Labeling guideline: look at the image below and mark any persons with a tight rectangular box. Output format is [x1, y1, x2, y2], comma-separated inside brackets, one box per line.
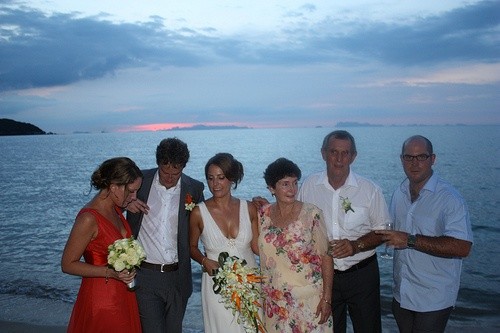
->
[62, 158, 143, 333]
[373, 134, 473, 333]
[188, 153, 264, 333]
[258, 158, 334, 333]
[122, 138, 206, 333]
[253, 130, 391, 333]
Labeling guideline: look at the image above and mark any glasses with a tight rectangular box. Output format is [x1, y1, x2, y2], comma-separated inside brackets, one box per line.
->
[402, 154, 433, 162]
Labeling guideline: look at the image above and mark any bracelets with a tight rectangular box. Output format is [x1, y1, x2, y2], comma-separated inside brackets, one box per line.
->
[324, 299, 332, 305]
[201, 256, 205, 271]
[353, 240, 362, 252]
[105, 269, 109, 282]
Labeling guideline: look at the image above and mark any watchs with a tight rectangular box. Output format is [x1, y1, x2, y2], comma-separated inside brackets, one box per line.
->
[407, 235, 416, 248]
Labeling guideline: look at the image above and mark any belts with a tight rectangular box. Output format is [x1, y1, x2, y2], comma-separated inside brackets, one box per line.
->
[334, 253, 377, 274]
[140, 261, 178, 273]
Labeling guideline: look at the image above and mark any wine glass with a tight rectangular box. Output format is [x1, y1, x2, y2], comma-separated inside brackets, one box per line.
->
[381, 222, 396, 259]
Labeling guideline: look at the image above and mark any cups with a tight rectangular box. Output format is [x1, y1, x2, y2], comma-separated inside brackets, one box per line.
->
[326, 233, 341, 256]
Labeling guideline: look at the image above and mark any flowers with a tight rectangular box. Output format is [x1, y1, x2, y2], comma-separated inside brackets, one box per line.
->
[185, 193, 195, 211]
[339, 196, 355, 214]
[211, 251, 266, 333]
[107, 236, 148, 291]
